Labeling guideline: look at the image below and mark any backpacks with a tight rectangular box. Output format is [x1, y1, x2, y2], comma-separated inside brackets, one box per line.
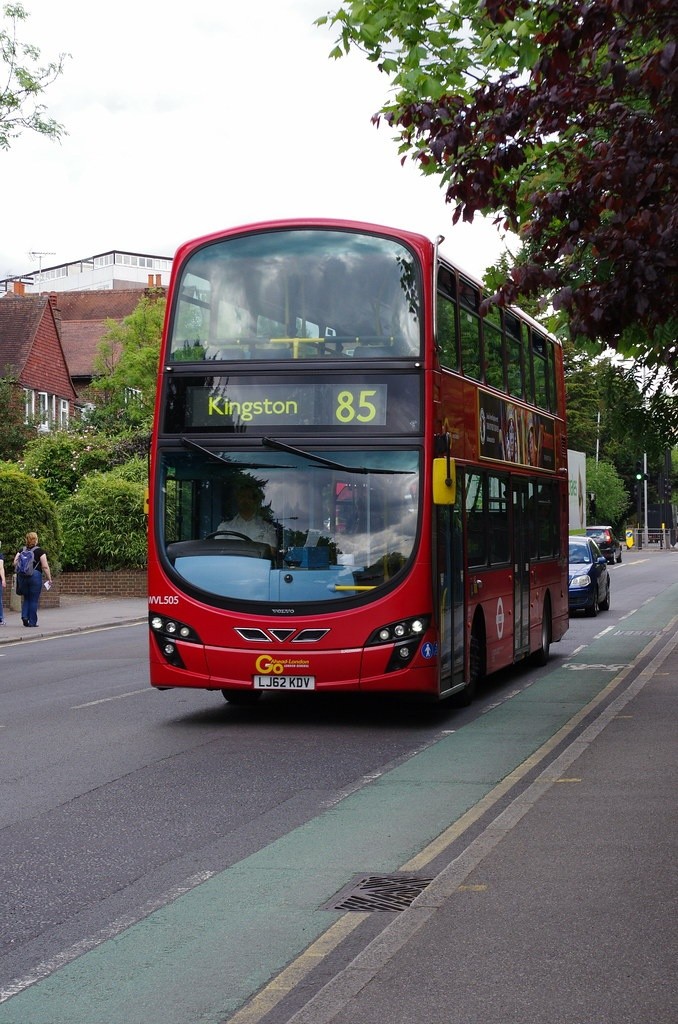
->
[14, 546, 42, 577]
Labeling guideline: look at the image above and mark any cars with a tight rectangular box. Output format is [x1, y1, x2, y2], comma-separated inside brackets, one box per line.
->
[568, 536, 611, 617]
[586, 526, 622, 565]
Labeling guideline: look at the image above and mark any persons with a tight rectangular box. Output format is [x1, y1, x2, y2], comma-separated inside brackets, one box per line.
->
[0, 541, 7, 625]
[528, 423, 536, 466]
[214, 483, 277, 559]
[14, 532, 52, 627]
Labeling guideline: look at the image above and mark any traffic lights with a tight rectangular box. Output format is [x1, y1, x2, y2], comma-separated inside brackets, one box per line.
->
[636, 473, 649, 481]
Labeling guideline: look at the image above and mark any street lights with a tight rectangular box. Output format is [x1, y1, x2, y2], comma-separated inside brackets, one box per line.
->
[590, 409, 613, 526]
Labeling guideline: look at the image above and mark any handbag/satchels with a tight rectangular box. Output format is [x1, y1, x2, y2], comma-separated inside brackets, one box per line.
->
[15, 572, 24, 596]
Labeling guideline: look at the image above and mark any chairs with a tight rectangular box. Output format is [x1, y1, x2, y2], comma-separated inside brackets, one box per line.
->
[214, 332, 409, 357]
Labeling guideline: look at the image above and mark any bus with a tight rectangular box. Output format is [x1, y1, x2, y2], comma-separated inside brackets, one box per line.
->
[147, 218, 568, 728]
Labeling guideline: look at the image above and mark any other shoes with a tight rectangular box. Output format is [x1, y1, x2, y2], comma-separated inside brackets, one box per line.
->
[23, 618, 28, 626]
[0, 622, 6, 627]
[30, 623, 39, 627]
